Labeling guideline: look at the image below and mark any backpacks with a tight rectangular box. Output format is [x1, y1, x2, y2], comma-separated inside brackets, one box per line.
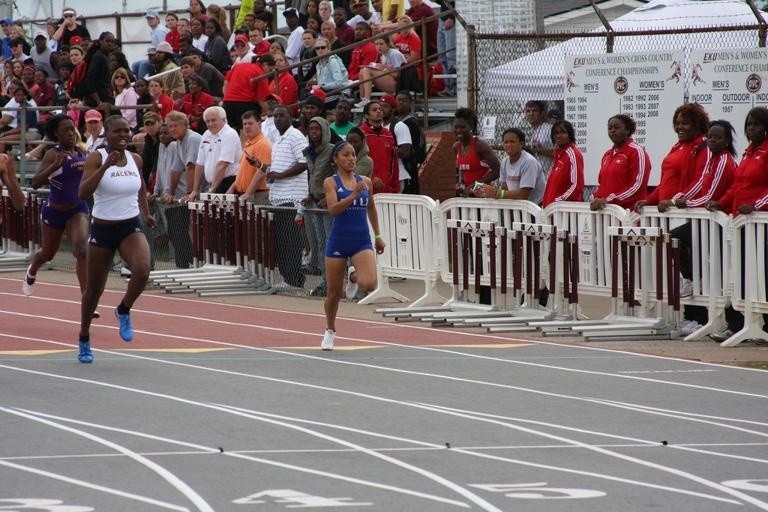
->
[403, 113, 427, 163]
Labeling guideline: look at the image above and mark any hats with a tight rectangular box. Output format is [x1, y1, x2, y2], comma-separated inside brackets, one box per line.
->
[63, 8, 76, 16]
[145, 41, 174, 60]
[303, 87, 327, 104]
[235, 35, 249, 46]
[143, 109, 161, 126]
[264, 93, 283, 108]
[234, 25, 249, 33]
[282, 6, 300, 21]
[84, 109, 103, 123]
[32, 30, 47, 41]
[144, 9, 159, 19]
[379, 94, 399, 110]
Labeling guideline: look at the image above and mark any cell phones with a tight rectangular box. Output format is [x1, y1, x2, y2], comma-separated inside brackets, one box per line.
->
[247, 157, 256, 164]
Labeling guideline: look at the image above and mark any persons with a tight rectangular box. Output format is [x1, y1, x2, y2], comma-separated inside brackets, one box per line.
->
[78, 115, 158, 361]
[21, 115, 102, 318]
[450, 99, 767, 346]
[316, 141, 387, 349]
[1, 0, 455, 296]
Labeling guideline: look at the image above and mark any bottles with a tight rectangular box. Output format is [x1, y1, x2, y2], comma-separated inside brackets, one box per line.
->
[294, 202, 305, 224]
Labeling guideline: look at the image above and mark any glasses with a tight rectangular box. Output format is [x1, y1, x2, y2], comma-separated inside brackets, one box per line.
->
[65, 14, 73, 18]
[114, 75, 125, 79]
[315, 45, 328, 50]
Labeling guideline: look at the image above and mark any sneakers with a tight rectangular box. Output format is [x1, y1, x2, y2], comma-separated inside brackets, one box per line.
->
[679, 278, 701, 299]
[345, 265, 360, 300]
[22, 264, 37, 295]
[271, 280, 304, 292]
[672, 318, 745, 348]
[320, 327, 336, 351]
[299, 261, 321, 277]
[77, 335, 94, 364]
[114, 304, 134, 342]
[119, 266, 132, 276]
[354, 96, 371, 111]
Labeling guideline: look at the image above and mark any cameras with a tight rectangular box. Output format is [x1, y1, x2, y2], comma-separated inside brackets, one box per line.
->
[473, 183, 483, 192]
